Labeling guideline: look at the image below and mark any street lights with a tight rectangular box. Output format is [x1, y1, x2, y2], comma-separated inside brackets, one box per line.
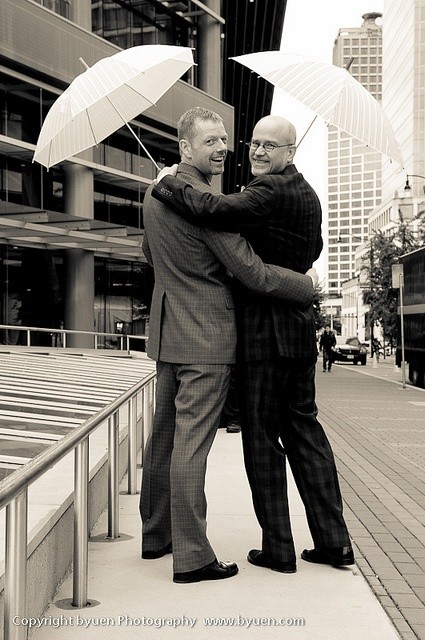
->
[337, 232, 374, 359]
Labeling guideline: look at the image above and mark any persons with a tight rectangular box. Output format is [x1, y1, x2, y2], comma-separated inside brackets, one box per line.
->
[139, 107, 324, 583]
[150, 114, 355, 574]
[319, 320, 337, 373]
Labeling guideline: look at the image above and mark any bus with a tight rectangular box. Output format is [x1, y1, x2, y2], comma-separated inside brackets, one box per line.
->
[393, 246, 424, 384]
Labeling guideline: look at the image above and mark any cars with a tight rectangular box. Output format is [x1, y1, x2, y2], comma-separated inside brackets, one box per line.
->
[332, 334, 368, 366]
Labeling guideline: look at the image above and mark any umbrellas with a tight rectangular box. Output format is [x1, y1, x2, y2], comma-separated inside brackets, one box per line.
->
[228, 45, 412, 172]
[29, 43, 198, 174]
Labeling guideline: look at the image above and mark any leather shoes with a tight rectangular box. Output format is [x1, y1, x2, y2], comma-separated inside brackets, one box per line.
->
[227, 422, 240, 432]
[247, 549, 296, 573]
[173, 560, 239, 583]
[301, 545, 354, 565]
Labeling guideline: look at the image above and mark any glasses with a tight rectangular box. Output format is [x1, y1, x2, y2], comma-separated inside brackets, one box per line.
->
[243, 142, 294, 151]
